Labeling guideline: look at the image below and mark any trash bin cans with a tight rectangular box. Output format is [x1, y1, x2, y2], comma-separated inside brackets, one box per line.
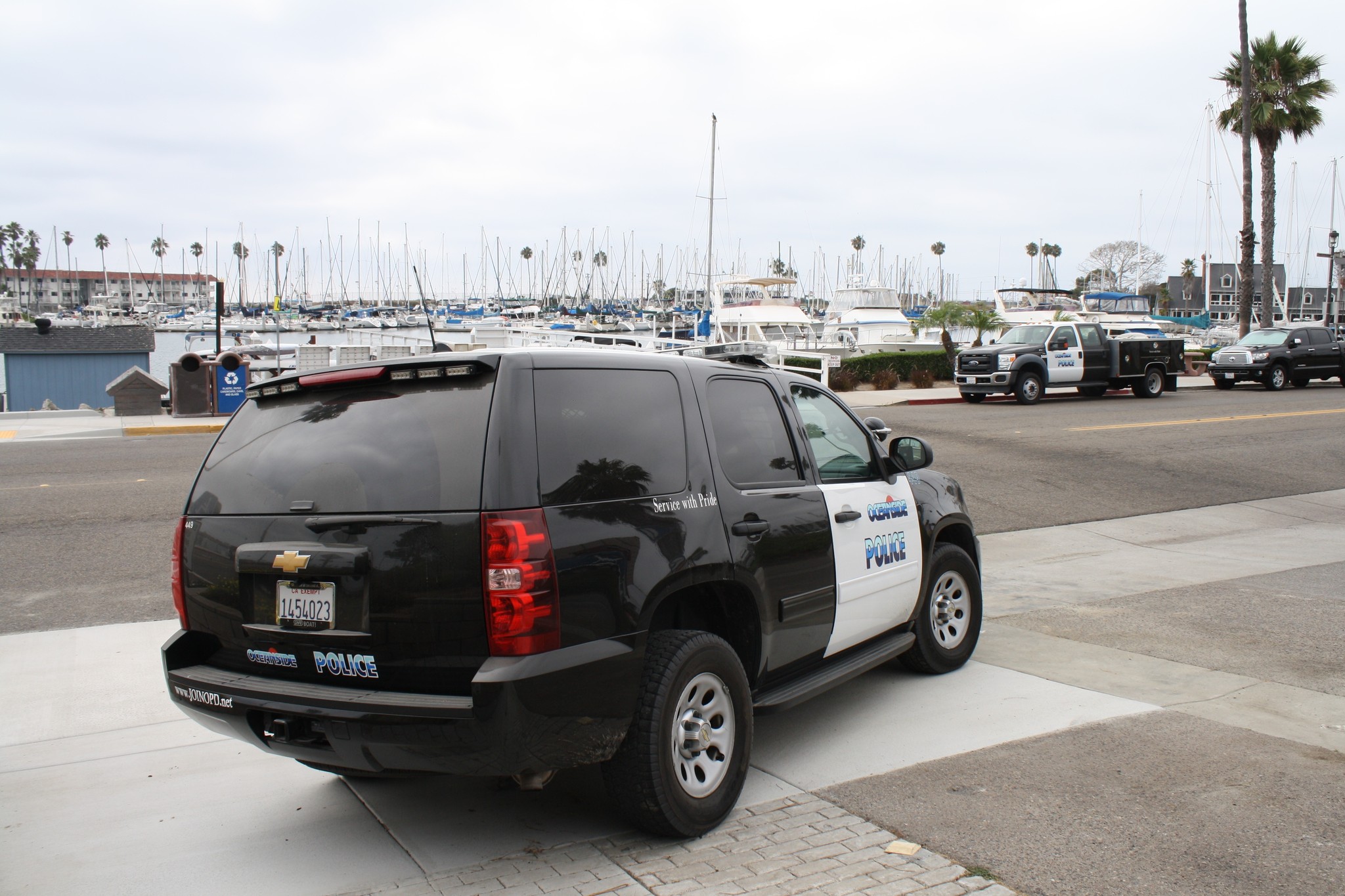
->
[210, 350, 251, 417]
[168, 352, 213, 418]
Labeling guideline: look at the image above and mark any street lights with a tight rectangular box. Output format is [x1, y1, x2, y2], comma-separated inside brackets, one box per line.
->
[1325, 230, 1340, 327]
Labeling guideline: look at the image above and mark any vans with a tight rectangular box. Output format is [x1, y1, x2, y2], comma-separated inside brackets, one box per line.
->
[160, 266, 983, 839]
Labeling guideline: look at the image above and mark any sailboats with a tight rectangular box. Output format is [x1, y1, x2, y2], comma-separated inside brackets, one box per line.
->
[0, 113, 957, 364]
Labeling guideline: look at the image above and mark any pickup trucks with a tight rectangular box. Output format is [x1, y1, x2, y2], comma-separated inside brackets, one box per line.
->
[1208, 327, 1345, 391]
[954, 321, 1189, 406]
